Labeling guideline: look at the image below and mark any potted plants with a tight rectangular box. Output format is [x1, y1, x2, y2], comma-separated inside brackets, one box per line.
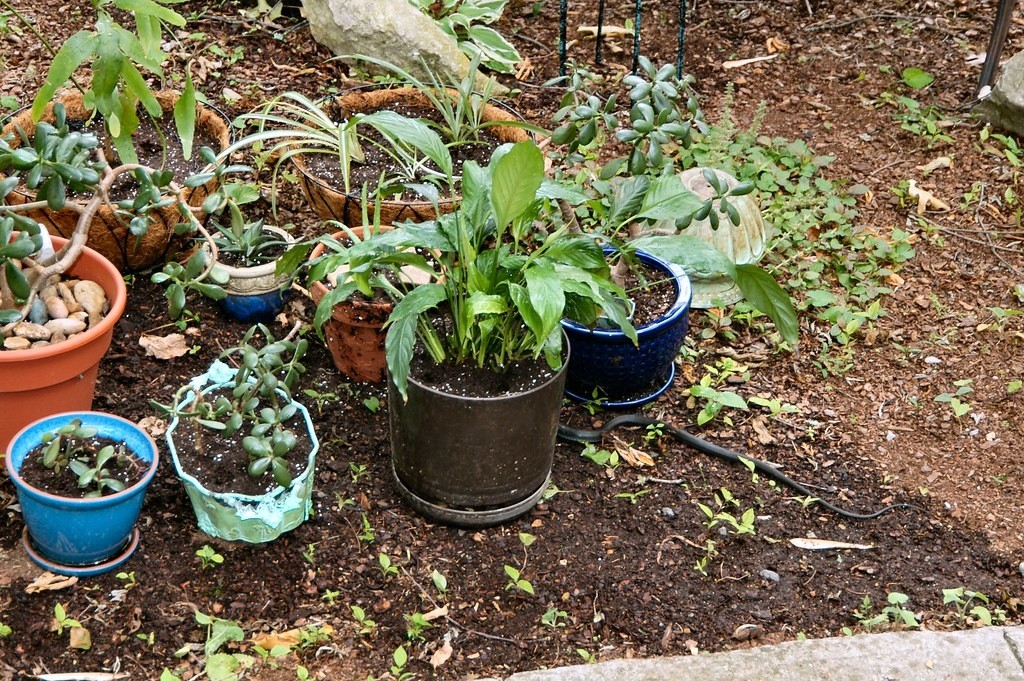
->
[192, 218, 306, 320]
[6, 410, 160, 577]
[197, 47, 552, 233]
[308, 138, 651, 528]
[306, 170, 448, 383]
[548, 52, 758, 414]
[148, 320, 321, 544]
[0, 1, 258, 339]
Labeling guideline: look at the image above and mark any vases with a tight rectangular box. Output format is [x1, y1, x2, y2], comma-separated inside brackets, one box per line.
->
[0, 229, 127, 463]
